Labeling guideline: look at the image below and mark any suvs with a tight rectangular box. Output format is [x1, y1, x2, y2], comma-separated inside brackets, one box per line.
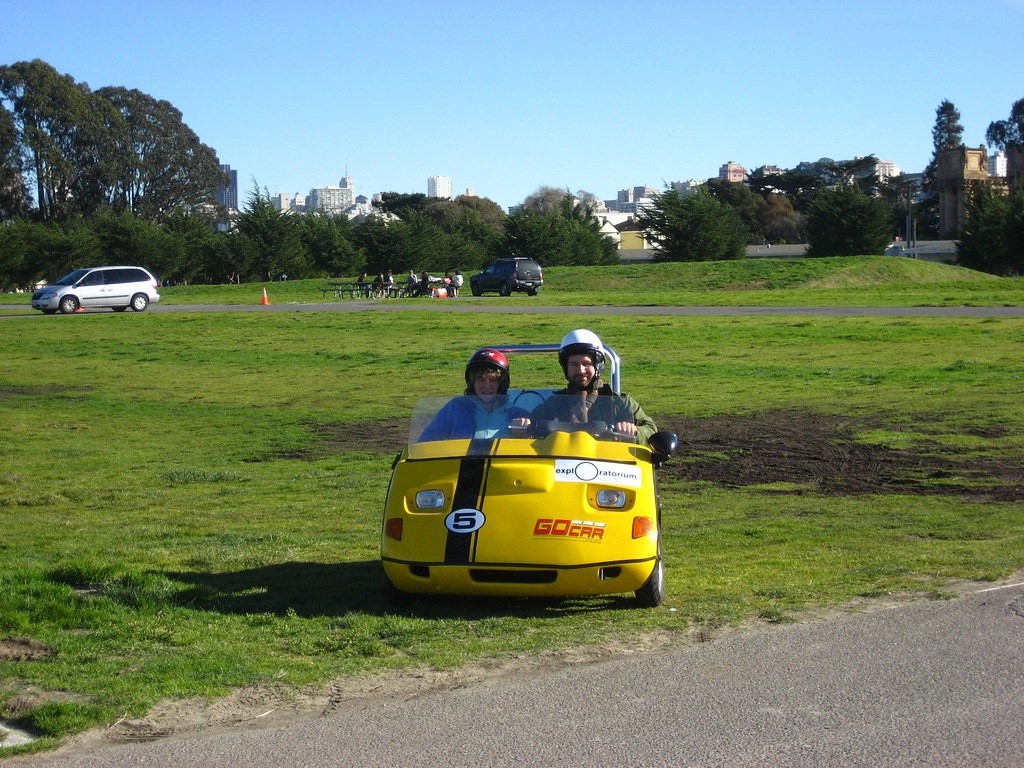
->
[469, 257, 544, 297]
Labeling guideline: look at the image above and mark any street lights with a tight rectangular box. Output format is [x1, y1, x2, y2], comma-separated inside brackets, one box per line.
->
[260, 287, 270, 306]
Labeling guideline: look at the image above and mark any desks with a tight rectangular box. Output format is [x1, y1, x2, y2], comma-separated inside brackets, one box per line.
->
[332, 282, 370, 299]
[397, 281, 442, 298]
[355, 282, 392, 298]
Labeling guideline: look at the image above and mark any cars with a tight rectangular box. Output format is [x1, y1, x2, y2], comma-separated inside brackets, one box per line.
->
[32, 265, 161, 314]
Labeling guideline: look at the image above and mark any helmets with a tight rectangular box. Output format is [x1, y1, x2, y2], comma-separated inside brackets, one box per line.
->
[466, 347, 509, 371]
[559, 329, 605, 363]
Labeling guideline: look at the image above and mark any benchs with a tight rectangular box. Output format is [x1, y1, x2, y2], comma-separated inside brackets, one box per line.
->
[322, 280, 458, 299]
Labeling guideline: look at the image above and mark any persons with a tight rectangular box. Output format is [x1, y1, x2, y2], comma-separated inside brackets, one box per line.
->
[417, 348, 531, 442]
[511, 329, 657, 446]
[358, 269, 463, 299]
